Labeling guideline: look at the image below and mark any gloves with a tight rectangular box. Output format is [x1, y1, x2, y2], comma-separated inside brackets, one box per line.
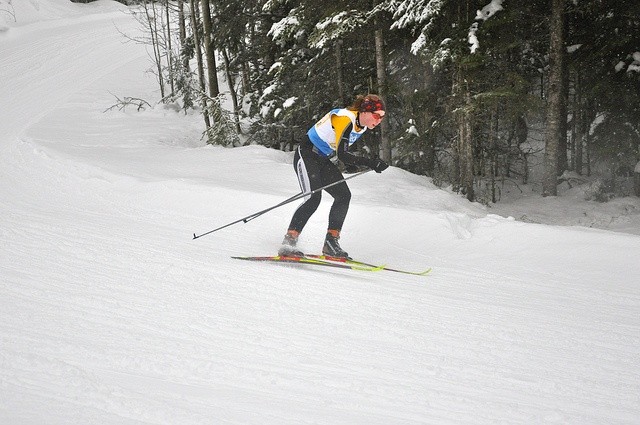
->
[370, 158, 389, 173]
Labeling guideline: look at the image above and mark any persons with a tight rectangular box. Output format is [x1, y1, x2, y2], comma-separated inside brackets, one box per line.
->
[279, 93, 390, 259]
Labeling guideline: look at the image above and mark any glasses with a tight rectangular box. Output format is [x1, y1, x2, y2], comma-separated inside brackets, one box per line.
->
[371, 110, 385, 119]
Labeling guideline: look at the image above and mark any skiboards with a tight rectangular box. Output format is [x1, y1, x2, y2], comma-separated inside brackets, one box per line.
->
[231, 251, 431, 276]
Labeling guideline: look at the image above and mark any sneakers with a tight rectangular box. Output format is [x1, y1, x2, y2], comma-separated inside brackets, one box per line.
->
[278, 234, 304, 257]
[322, 232, 348, 257]
